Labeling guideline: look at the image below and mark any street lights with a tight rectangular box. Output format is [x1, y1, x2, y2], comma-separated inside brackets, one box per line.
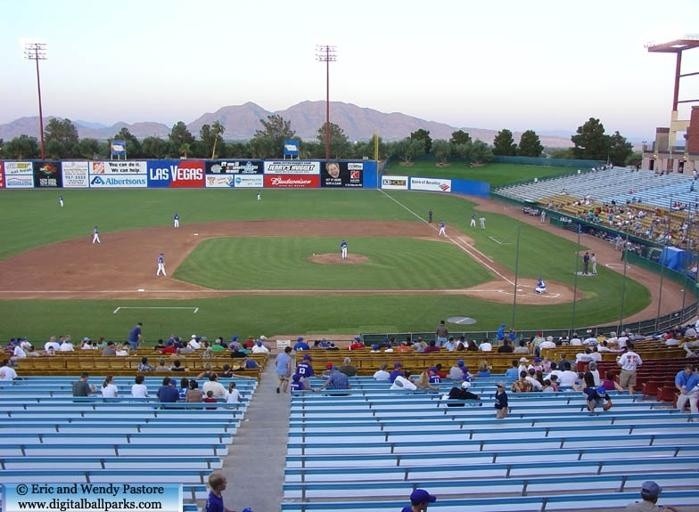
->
[607, 144, 614, 163]
[23, 40, 48, 159]
[315, 44, 338, 158]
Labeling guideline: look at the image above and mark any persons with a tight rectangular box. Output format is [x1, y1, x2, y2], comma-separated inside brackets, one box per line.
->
[58, 195, 65, 207]
[469, 214, 477, 227]
[92, 225, 102, 245]
[437, 220, 447, 238]
[172, 212, 181, 229]
[325, 162, 340, 179]
[521, 167, 699, 276]
[400, 488, 437, 511]
[156, 252, 168, 277]
[340, 239, 349, 260]
[535, 277, 546, 294]
[478, 216, 487, 231]
[626, 480, 676, 511]
[203, 473, 240, 511]
[255, 190, 262, 201]
[427, 207, 434, 224]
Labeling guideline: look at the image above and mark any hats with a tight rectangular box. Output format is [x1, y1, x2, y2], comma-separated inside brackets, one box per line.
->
[461, 381, 471, 389]
[293, 374, 301, 379]
[326, 362, 333, 369]
[640, 480, 662, 496]
[304, 354, 312, 361]
[596, 387, 606, 398]
[458, 360, 464, 367]
[409, 489, 437, 502]
[394, 362, 403, 369]
[520, 357, 529, 363]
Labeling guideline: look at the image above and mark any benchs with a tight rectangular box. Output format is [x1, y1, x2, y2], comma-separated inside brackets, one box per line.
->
[490, 164, 699, 258]
[1, 332, 699, 511]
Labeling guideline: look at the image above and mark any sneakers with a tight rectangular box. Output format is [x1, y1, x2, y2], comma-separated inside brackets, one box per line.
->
[277, 387, 280, 394]
